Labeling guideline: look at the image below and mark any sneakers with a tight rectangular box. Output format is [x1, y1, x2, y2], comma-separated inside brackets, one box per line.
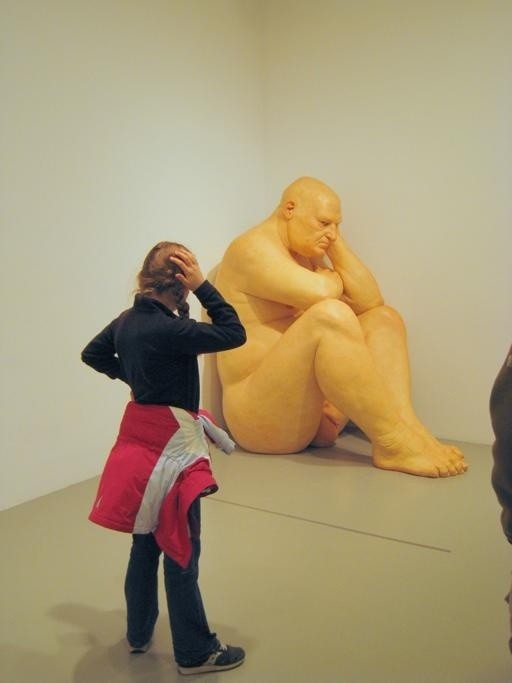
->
[127, 636, 154, 653]
[178, 644, 245, 675]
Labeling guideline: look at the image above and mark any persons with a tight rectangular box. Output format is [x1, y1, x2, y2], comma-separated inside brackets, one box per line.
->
[216, 176, 468, 477]
[489, 347, 512, 655]
[79, 241, 246, 677]
